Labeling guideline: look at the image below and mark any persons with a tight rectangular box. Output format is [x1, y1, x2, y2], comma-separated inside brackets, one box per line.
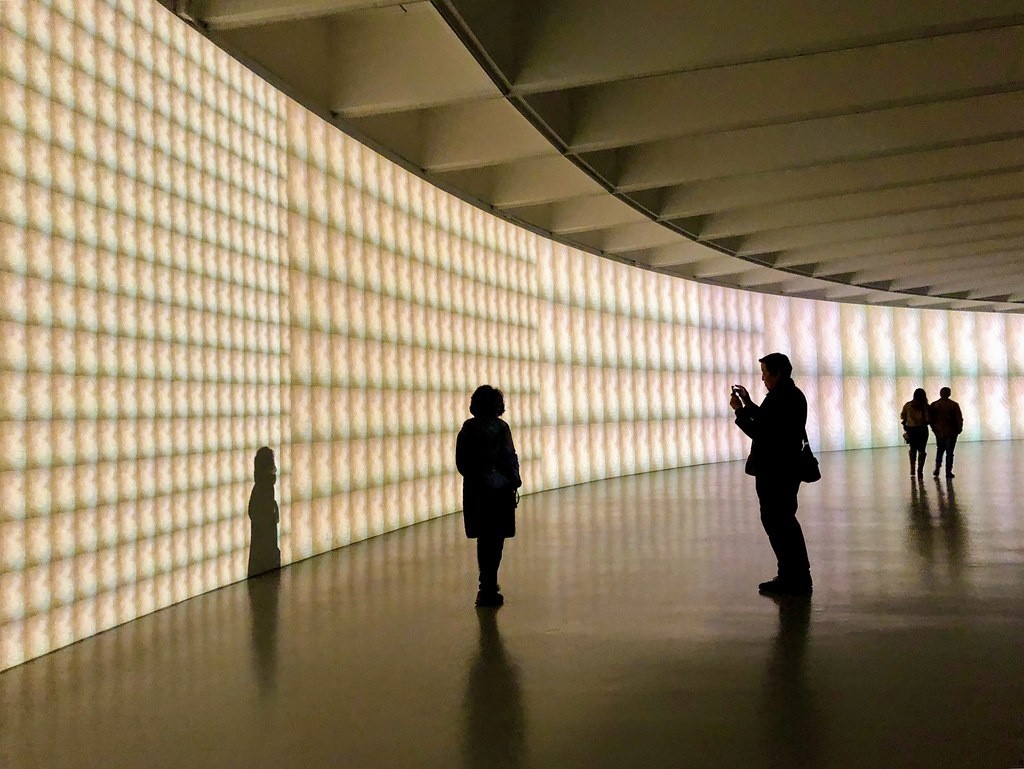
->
[928, 386, 964, 479]
[456, 384, 523, 611]
[900, 388, 930, 478]
[730, 352, 813, 596]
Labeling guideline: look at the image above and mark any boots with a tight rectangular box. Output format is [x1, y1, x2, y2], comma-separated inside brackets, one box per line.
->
[909, 450, 916, 475]
[918, 453, 926, 478]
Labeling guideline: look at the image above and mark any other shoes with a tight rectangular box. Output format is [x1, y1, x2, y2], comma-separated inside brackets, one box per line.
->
[934, 470, 939, 476]
[759, 576, 812, 594]
[946, 473, 955, 478]
[474, 593, 503, 606]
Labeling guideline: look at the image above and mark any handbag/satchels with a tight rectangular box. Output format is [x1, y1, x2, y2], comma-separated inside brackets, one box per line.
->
[802, 444, 821, 482]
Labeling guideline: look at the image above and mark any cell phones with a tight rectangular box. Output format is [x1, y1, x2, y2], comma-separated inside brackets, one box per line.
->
[731, 386, 736, 395]
